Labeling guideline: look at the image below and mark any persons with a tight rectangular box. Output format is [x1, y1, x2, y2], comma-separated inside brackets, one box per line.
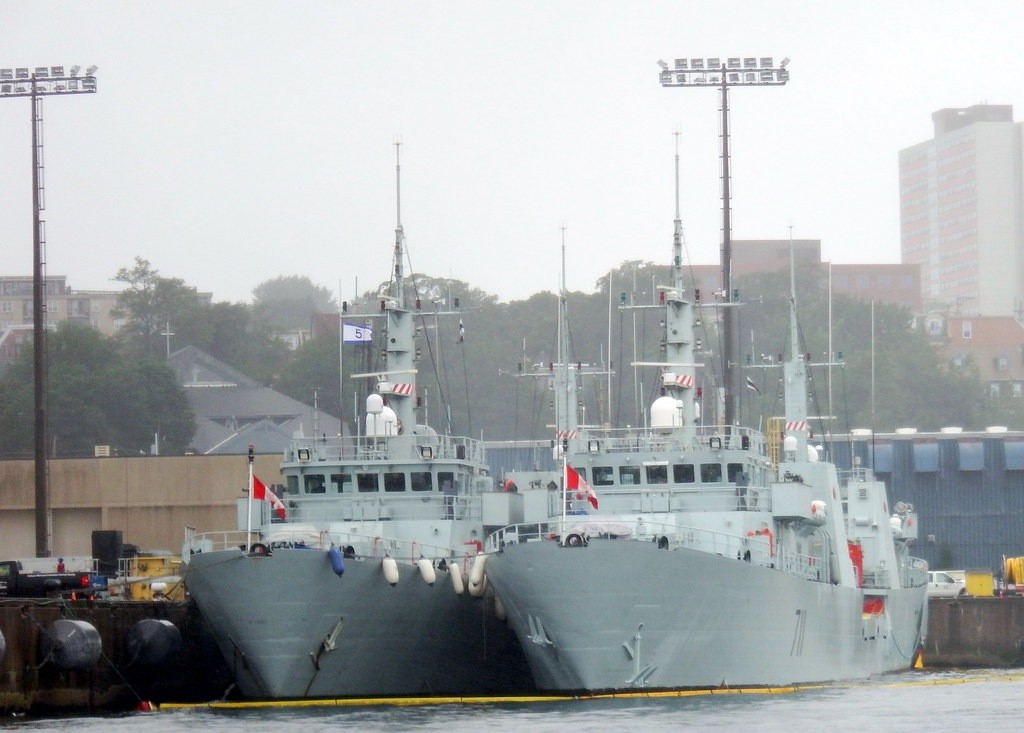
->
[496, 476, 514, 492]
[464, 530, 482, 554]
[57, 557, 65, 575]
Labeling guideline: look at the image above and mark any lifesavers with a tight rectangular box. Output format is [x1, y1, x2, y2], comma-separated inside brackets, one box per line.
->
[566, 534, 582, 546]
[745, 550, 751, 561]
[658, 536, 669, 550]
[344, 546, 355, 558]
[249, 543, 268, 557]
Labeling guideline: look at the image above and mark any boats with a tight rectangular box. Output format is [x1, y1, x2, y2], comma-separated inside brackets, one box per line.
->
[179, 127, 532, 710]
[481, 125, 931, 704]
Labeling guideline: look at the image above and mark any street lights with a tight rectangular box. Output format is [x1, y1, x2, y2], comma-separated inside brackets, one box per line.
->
[658, 56, 793, 441]
[0, 61, 98, 562]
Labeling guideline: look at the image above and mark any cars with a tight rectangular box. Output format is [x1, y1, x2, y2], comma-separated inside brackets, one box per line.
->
[946, 568, 1024, 597]
[927, 570, 970, 597]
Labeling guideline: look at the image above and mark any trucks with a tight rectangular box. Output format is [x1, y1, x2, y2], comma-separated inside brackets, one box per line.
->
[0, 560, 98, 604]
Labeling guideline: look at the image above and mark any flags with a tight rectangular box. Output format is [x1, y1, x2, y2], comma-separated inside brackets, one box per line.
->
[343, 321, 374, 345]
[253, 476, 286, 521]
[567, 466, 599, 510]
